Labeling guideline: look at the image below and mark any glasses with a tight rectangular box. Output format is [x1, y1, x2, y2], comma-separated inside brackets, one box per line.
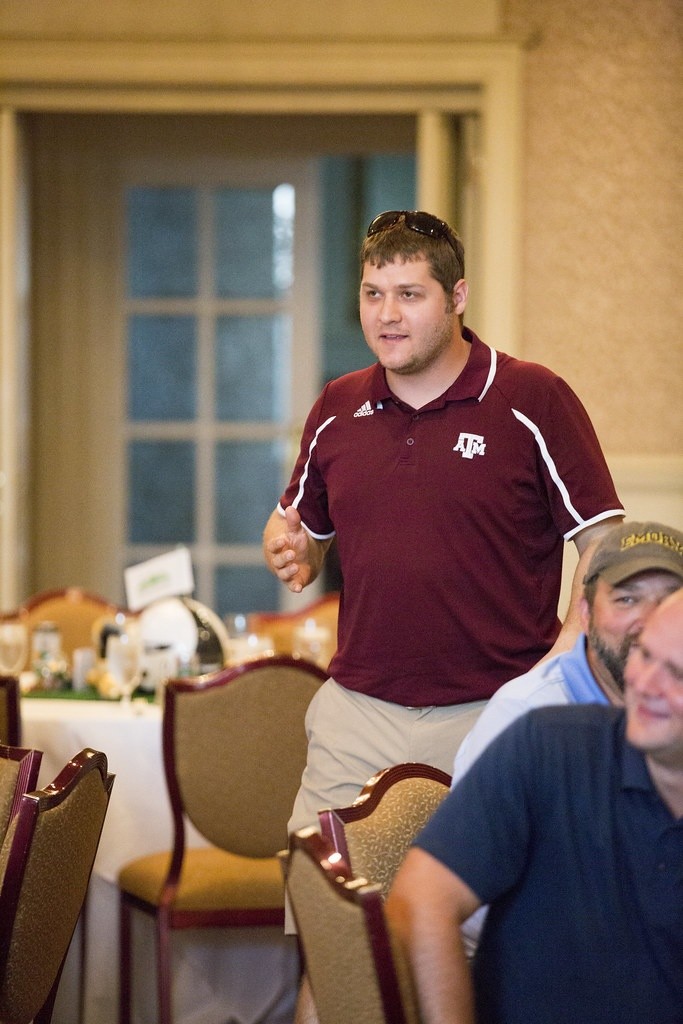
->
[366, 209, 460, 265]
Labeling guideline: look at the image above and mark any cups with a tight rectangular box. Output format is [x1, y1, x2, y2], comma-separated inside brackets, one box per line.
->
[0, 624, 29, 677]
[72, 649, 95, 692]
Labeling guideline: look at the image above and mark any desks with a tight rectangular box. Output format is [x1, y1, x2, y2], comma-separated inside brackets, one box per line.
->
[14, 696, 299, 1024]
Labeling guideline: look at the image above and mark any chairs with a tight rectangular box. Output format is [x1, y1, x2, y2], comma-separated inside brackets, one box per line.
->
[0, 588, 452, 1024]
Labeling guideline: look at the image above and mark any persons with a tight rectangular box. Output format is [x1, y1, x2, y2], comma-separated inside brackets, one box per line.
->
[260, 210, 629, 1024]
[447, 522, 683, 958]
[384, 587, 683, 1024]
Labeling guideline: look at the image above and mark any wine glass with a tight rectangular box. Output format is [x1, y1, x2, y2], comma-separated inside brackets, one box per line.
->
[105, 636, 143, 709]
[31, 623, 63, 689]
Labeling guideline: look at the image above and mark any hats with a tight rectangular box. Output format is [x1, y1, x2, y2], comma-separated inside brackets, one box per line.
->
[582, 521, 683, 588]
